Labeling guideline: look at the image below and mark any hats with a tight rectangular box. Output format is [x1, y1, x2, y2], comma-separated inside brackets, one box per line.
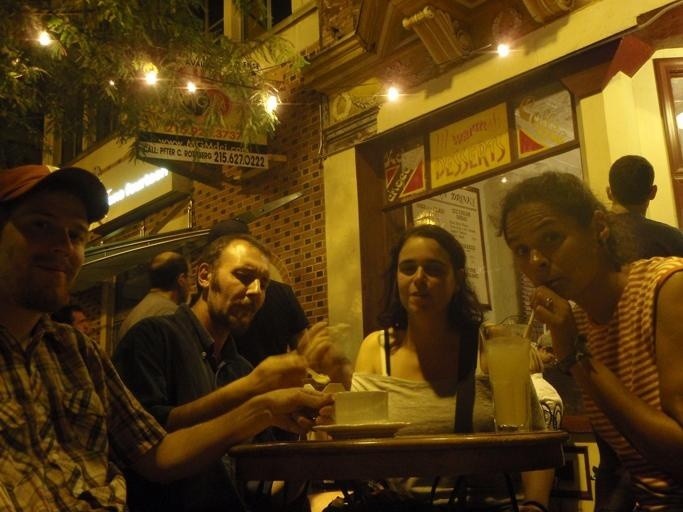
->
[0, 164, 108, 223]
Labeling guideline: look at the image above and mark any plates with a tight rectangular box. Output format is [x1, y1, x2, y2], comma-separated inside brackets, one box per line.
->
[312, 423, 411, 440]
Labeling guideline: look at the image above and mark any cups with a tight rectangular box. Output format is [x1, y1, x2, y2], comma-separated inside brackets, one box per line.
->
[480, 325, 535, 433]
[333, 391, 388, 424]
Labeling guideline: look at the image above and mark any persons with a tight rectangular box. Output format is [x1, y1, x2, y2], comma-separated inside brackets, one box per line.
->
[605, 156, 682, 272]
[349, 226, 556, 512]
[495, 171, 682, 512]
[50, 220, 357, 512]
[2, 165, 336, 512]
